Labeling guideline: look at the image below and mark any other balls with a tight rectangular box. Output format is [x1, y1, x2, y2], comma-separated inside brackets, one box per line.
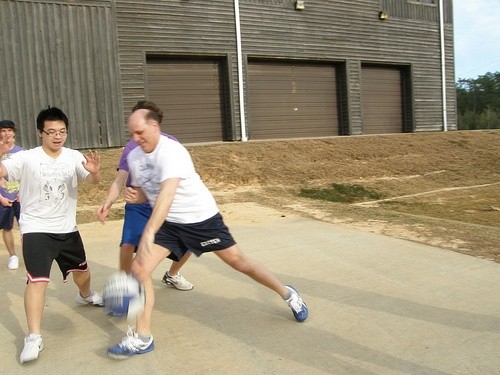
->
[101, 270, 148, 322]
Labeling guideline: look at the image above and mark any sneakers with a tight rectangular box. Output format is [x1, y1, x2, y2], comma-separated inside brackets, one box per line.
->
[162, 271, 194, 290]
[76, 291, 104, 307]
[284, 285, 309, 322]
[20, 334, 44, 363]
[107, 324, 155, 359]
[8, 256, 19, 270]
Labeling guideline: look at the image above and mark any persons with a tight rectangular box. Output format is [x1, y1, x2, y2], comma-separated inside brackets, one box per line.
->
[0, 107, 101, 366]
[97, 101, 308, 361]
[0, 120, 26, 270]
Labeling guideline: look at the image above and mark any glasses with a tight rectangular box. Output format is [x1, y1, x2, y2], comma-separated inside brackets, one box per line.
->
[42, 129, 67, 138]
